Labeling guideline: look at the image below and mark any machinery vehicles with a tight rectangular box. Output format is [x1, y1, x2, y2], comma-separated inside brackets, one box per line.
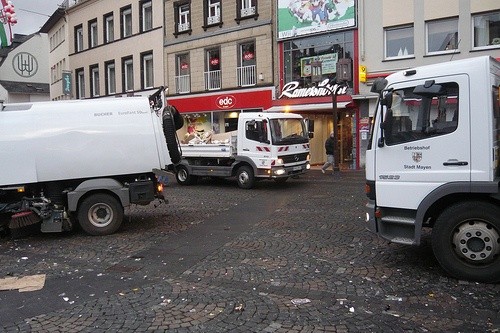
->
[0, 86, 184, 236]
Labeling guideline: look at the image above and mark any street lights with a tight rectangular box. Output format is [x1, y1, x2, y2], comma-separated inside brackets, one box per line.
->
[310, 58, 351, 179]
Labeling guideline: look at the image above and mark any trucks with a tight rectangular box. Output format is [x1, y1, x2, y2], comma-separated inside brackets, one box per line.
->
[363, 55, 500, 283]
[177, 112, 314, 190]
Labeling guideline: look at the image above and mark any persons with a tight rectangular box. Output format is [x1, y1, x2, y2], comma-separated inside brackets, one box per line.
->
[321, 131, 336, 175]
